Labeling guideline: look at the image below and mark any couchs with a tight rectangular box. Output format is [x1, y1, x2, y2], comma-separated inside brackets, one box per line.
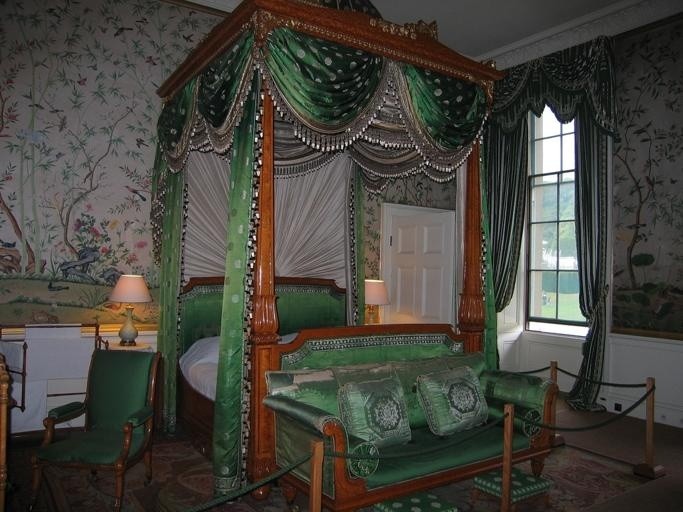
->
[262, 321, 559, 512]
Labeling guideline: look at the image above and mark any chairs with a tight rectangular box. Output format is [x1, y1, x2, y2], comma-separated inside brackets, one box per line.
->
[24, 347, 162, 511]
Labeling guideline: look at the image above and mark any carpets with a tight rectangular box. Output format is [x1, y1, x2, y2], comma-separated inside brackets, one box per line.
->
[43, 439, 648, 512]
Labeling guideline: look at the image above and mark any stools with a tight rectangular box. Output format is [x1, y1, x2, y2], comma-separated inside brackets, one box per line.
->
[370, 466, 553, 512]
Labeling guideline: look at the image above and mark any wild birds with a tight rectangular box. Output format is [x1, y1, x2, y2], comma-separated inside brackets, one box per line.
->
[0, 239, 18, 248]
[135, 137, 151, 149]
[145, 55, 158, 67]
[624, 223, 647, 231]
[113, 18, 149, 39]
[183, 34, 195, 44]
[123, 184, 147, 203]
[632, 177, 658, 201]
[48, 283, 69, 291]
[20, 89, 66, 114]
[663, 283, 683, 305]
[77, 73, 88, 86]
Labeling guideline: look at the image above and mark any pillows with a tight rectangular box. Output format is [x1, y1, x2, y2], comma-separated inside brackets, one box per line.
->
[265, 351, 488, 449]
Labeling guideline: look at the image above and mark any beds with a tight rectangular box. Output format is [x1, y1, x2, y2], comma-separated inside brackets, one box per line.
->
[176, 275, 464, 500]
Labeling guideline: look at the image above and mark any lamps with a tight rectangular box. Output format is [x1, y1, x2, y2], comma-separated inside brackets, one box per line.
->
[109, 273, 153, 346]
[364, 279, 390, 324]
